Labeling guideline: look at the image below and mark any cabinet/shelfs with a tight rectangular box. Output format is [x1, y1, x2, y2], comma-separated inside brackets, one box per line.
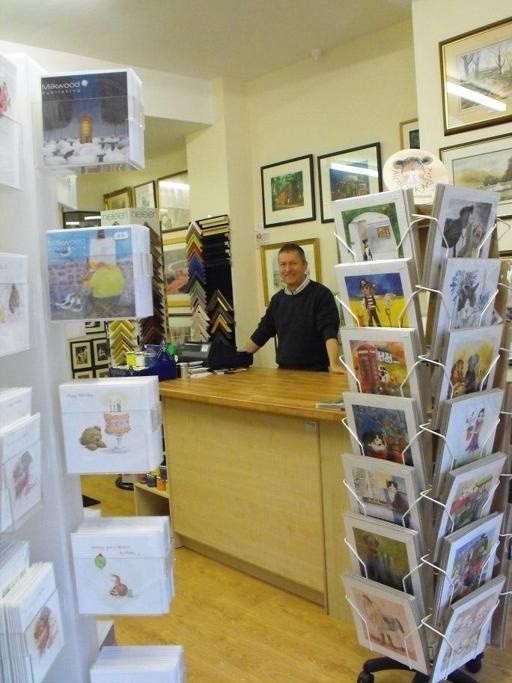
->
[130, 401, 185, 550]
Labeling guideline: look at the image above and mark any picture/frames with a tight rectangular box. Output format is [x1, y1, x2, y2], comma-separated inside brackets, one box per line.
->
[438, 132, 511, 221]
[438, 15, 512, 137]
[168, 312, 194, 345]
[162, 237, 191, 306]
[399, 117, 418, 151]
[259, 152, 315, 228]
[70, 320, 111, 381]
[133, 181, 156, 209]
[156, 169, 195, 234]
[259, 238, 322, 307]
[316, 141, 383, 224]
[102, 185, 132, 210]
[496, 250, 512, 368]
